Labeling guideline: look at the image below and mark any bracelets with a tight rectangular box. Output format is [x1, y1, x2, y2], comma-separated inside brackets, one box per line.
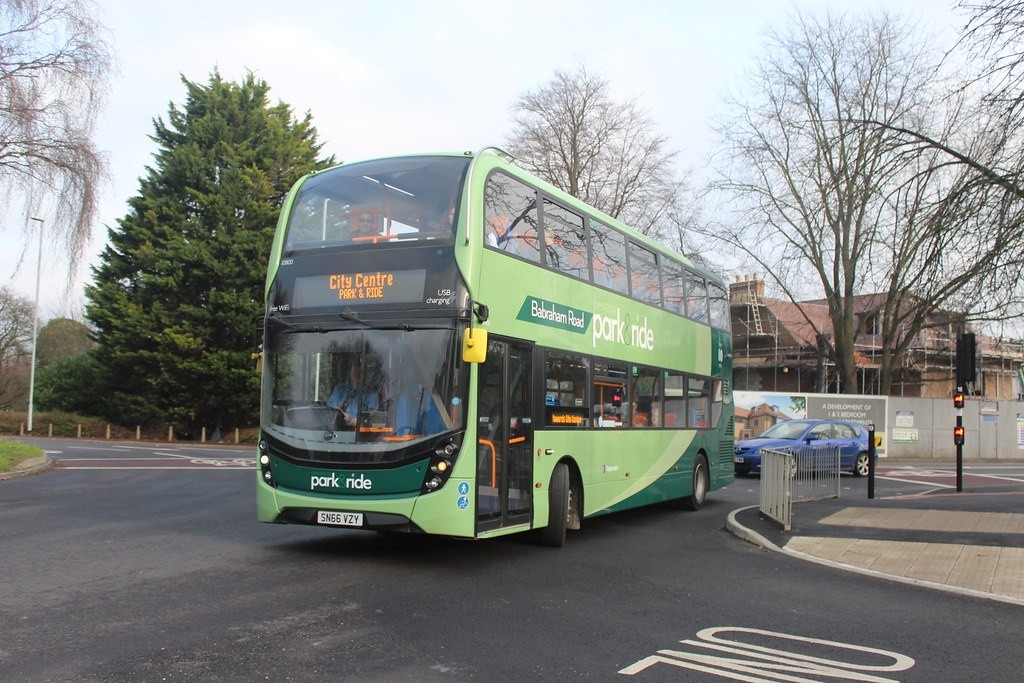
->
[346, 414, 353, 425]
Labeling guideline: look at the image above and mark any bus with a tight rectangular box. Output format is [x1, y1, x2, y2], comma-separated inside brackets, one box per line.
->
[251, 146, 734, 547]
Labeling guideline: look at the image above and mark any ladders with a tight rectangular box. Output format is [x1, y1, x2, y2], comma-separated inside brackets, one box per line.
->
[769, 314, 786, 362]
[750, 291, 762, 333]
[966, 382, 975, 396]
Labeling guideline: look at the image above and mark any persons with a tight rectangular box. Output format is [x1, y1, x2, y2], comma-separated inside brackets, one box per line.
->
[348, 212, 387, 243]
[328, 360, 380, 432]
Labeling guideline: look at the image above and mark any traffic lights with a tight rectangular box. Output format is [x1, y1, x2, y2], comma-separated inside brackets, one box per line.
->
[954, 427, 963, 446]
[954, 394, 964, 408]
[612, 390, 621, 407]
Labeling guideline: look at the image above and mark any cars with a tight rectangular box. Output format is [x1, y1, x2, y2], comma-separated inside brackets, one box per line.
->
[734, 419, 878, 479]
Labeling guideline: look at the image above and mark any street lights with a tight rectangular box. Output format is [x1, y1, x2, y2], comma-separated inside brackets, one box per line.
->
[25, 217, 44, 433]
